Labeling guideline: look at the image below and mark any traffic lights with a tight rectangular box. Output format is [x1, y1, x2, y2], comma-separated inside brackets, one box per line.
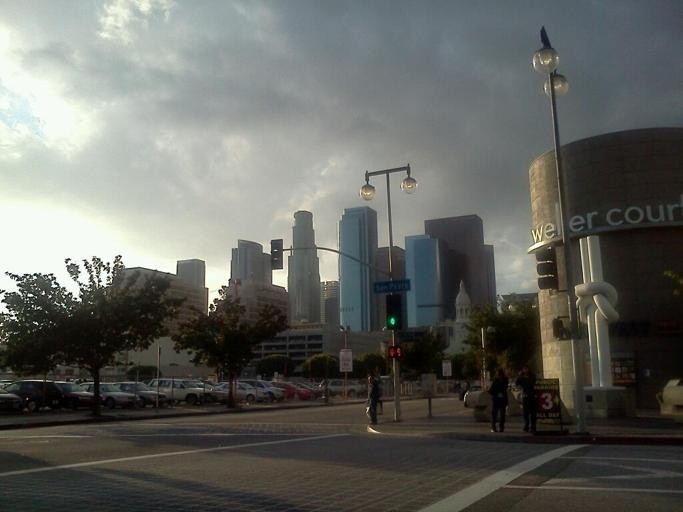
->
[557, 327, 571, 340]
[385, 293, 402, 331]
[388, 345, 403, 359]
[270, 237, 284, 271]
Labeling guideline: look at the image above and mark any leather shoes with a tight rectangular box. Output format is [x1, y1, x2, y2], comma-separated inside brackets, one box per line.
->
[498, 423, 503, 432]
[490, 424, 496, 432]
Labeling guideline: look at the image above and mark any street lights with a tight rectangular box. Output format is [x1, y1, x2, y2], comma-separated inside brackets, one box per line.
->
[531, 24, 590, 443]
[339, 324, 350, 399]
[358, 162, 418, 424]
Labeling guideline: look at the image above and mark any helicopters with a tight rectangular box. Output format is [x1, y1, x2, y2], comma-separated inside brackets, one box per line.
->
[534, 244, 560, 292]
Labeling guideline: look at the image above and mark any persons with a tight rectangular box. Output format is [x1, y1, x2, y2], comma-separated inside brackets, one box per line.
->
[515, 363, 537, 432]
[486, 367, 509, 432]
[463, 378, 469, 392]
[368, 375, 381, 425]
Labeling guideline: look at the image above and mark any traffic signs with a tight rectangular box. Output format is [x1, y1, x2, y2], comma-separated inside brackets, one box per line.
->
[373, 279, 411, 293]
